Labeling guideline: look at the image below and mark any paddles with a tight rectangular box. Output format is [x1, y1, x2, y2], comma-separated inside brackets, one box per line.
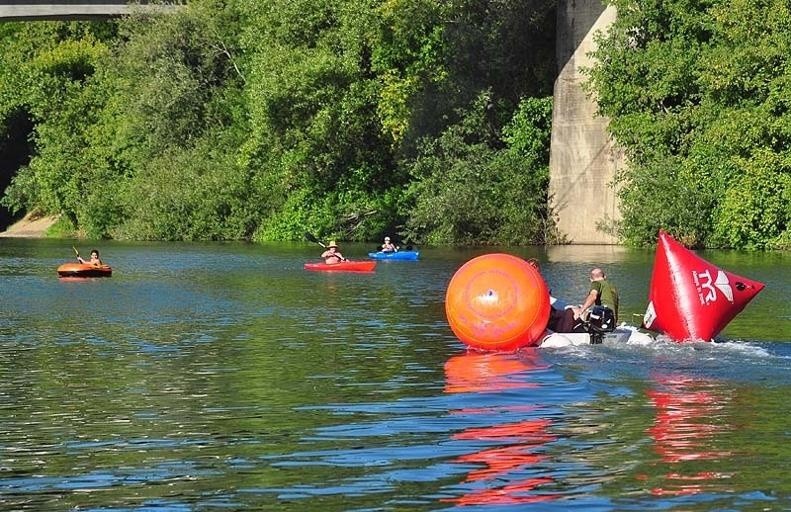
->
[317, 242, 350, 262]
[73, 246, 82, 264]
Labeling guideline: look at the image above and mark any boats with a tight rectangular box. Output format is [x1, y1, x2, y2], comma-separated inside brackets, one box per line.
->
[304, 260, 377, 272]
[58, 260, 113, 279]
[534, 294, 657, 351]
[370, 249, 420, 262]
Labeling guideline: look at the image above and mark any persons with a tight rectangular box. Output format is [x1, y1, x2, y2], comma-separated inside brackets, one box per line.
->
[559, 267, 620, 333]
[382, 236, 398, 252]
[78, 250, 102, 266]
[321, 241, 349, 264]
[529, 256, 566, 333]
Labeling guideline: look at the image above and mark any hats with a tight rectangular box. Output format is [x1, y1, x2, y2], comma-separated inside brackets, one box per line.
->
[326, 241, 338, 248]
[384, 236, 390, 241]
